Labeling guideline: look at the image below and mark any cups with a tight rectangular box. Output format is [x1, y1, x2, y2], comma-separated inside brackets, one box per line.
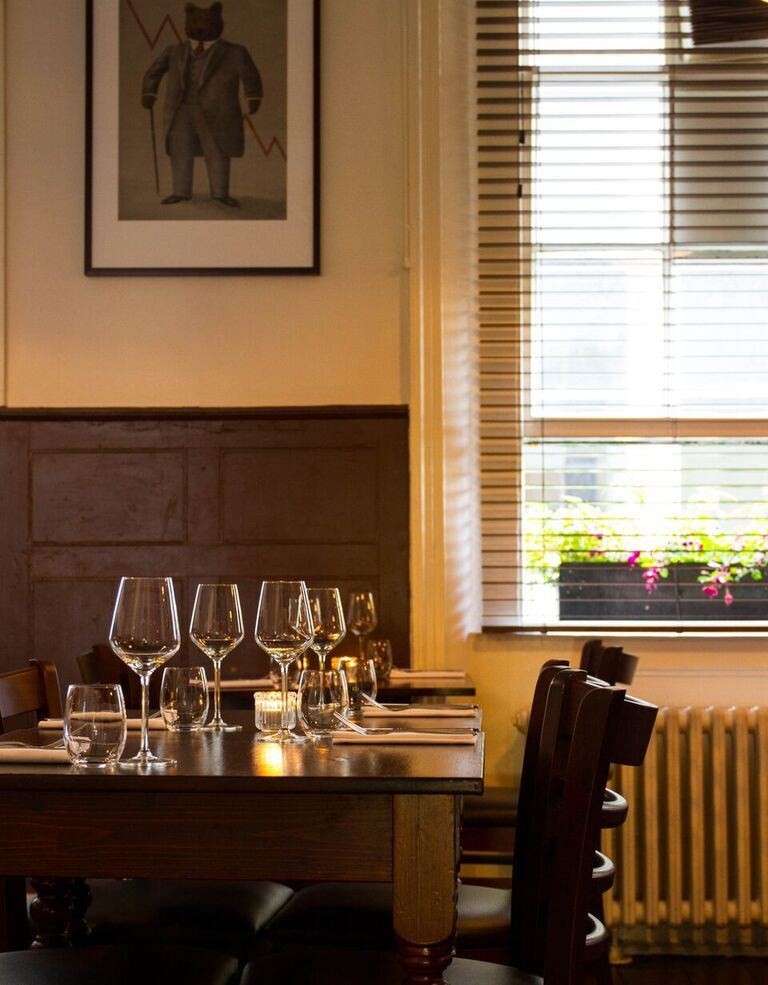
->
[367, 638, 393, 678]
[253, 691, 298, 735]
[63, 684, 127, 768]
[159, 666, 210, 733]
[337, 658, 378, 706]
[296, 668, 349, 735]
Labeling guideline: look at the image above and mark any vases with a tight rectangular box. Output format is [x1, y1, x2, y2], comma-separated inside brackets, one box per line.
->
[559, 561, 768, 620]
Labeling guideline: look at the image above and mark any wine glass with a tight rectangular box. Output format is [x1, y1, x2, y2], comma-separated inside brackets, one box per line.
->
[347, 592, 377, 661]
[254, 581, 315, 744]
[188, 583, 245, 732]
[296, 588, 347, 712]
[108, 575, 181, 769]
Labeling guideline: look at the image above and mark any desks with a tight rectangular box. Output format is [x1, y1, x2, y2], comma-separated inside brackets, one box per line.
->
[181, 670, 476, 705]
[0, 704, 485, 985]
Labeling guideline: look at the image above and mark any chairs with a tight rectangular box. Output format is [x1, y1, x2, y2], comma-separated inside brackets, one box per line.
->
[0, 642, 658, 985]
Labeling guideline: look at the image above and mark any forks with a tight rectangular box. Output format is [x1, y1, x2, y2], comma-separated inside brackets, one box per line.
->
[0, 723, 83, 749]
[332, 693, 473, 736]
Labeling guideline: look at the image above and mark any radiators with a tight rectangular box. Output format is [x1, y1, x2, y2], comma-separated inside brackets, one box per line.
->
[516, 703, 768, 964]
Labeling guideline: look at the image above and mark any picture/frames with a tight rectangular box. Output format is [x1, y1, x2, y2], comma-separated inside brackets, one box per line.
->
[83, 0, 321, 278]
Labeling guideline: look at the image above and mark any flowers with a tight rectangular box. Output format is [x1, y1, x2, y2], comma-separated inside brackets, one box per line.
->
[528, 490, 768, 606]
